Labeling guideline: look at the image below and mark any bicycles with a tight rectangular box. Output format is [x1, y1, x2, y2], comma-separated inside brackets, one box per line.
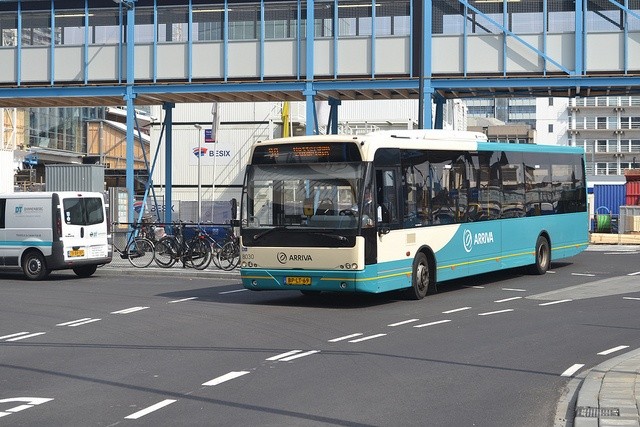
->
[140, 217, 241, 272]
[97, 220, 156, 268]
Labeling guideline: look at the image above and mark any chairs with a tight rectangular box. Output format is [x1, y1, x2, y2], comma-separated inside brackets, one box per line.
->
[406, 183, 563, 225]
[315, 198, 334, 214]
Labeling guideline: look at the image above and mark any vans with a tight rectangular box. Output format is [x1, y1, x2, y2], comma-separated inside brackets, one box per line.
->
[1, 190, 113, 281]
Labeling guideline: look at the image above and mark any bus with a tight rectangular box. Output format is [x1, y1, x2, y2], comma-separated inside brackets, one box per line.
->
[238, 128, 591, 300]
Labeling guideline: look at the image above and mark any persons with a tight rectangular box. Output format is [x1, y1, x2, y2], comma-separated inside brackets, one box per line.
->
[352, 188, 383, 222]
